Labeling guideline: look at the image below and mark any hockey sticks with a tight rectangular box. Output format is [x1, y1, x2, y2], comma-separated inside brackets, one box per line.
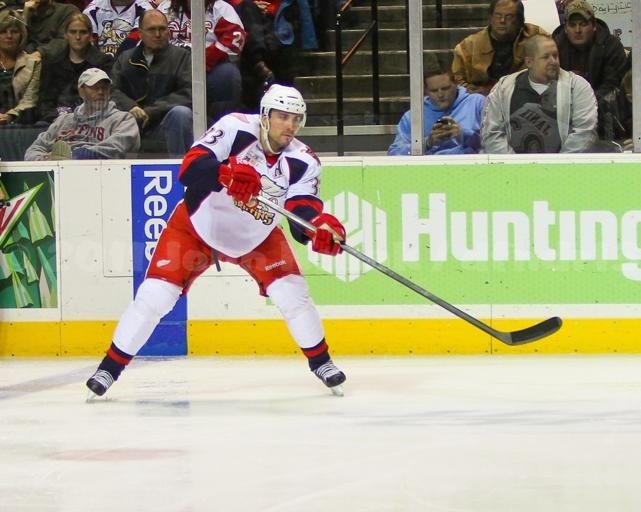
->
[258, 197, 562, 345]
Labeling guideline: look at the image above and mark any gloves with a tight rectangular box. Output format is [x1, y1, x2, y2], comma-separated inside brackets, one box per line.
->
[305, 213, 345, 256]
[218, 156, 261, 208]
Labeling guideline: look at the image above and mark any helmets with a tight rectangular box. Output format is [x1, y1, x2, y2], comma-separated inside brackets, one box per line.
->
[260, 84, 307, 132]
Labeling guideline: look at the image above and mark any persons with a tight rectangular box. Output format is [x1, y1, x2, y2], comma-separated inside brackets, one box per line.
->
[0, 0, 348, 159]
[80, 84, 346, 357]
[385, 0, 633, 153]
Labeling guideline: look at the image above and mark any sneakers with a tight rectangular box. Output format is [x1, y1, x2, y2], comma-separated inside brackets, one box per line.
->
[313, 359, 346, 387]
[87, 369, 114, 395]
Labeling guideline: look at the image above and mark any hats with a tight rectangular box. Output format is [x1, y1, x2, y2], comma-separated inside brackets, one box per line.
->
[77, 68, 112, 88]
[564, 0, 594, 21]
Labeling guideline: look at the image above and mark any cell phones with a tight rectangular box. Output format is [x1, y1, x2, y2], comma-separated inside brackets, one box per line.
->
[437, 119, 449, 125]
